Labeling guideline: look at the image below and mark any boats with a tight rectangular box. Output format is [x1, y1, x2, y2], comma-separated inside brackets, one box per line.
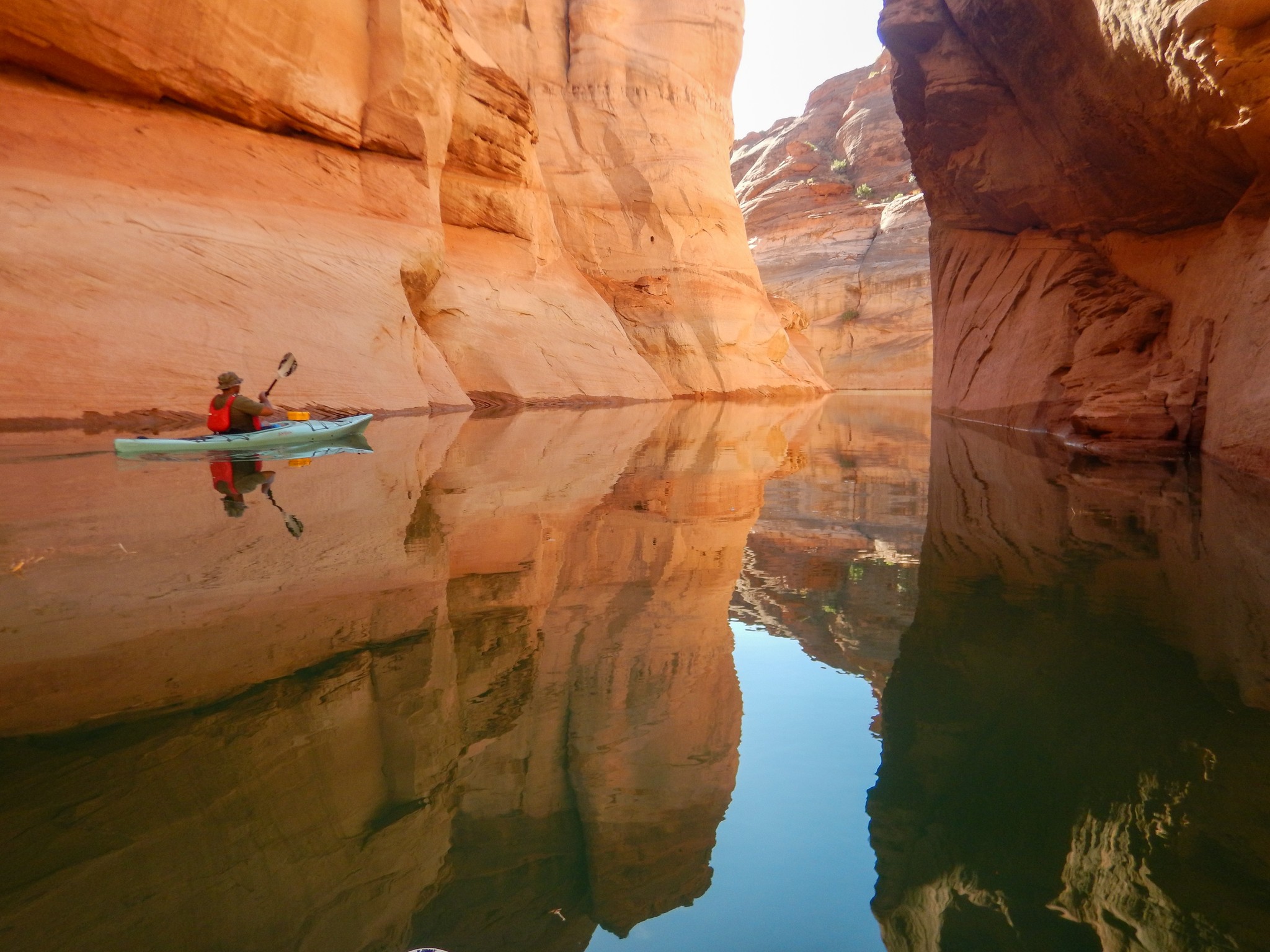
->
[118, 433, 375, 462]
[112, 412, 374, 454]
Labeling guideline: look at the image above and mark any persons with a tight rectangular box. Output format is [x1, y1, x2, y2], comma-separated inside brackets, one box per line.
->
[214, 371, 274, 435]
[209, 461, 276, 518]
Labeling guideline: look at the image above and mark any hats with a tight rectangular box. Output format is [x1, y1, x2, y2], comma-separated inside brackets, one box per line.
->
[216, 372, 243, 389]
[220, 498, 249, 518]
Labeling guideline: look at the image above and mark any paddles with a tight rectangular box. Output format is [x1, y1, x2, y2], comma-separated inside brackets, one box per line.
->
[260, 352, 298, 403]
[267, 489, 304, 540]
